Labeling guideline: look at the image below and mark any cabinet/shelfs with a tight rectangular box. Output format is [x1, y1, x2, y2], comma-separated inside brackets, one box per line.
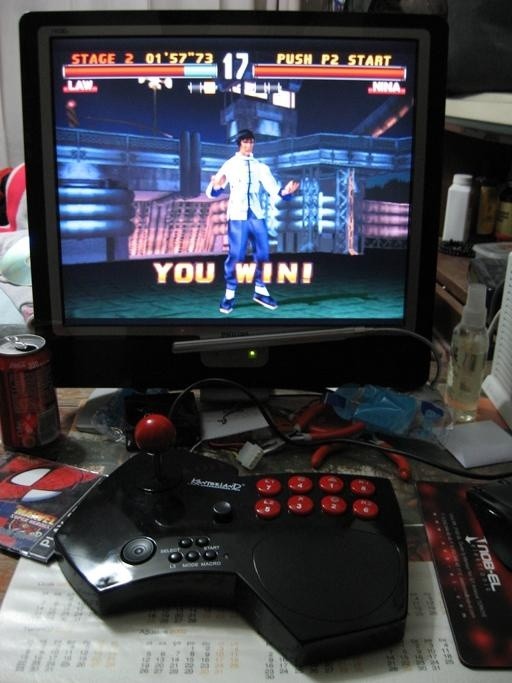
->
[433, 0, 512, 347]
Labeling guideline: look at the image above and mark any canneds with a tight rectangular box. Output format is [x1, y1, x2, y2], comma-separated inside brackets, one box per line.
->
[0, 334, 60, 454]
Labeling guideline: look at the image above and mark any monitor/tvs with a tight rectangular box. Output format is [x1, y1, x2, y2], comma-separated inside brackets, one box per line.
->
[18, 10, 452, 435]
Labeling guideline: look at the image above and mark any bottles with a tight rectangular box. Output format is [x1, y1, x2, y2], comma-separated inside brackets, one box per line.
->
[444, 282, 490, 424]
[439, 173, 512, 244]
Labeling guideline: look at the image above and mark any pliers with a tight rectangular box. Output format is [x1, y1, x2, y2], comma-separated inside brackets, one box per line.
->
[261, 400, 412, 482]
